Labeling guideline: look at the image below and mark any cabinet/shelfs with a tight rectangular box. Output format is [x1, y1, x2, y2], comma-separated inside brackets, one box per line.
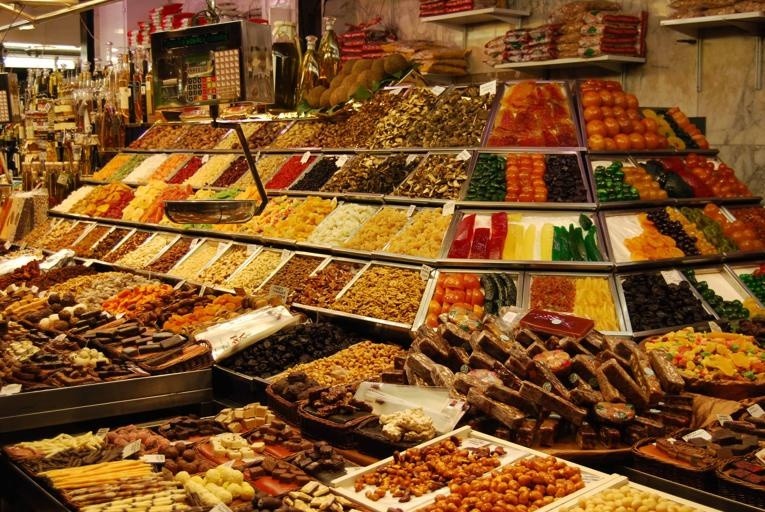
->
[0, 49, 145, 244]
[0, 82, 765, 512]
[419, 7, 760, 93]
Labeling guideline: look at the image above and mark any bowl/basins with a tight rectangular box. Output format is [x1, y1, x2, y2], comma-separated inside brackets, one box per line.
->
[161, 199, 258, 223]
[162, 110, 183, 121]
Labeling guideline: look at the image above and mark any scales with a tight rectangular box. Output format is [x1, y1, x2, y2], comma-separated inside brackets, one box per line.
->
[151, 21, 269, 225]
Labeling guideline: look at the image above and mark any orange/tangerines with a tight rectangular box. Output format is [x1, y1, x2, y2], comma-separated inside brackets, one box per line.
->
[613, 106, 626, 118]
[629, 133, 645, 150]
[631, 119, 645, 134]
[644, 132, 658, 149]
[612, 91, 627, 109]
[588, 134, 606, 151]
[625, 94, 639, 109]
[600, 89, 614, 107]
[582, 92, 601, 109]
[657, 135, 668, 149]
[587, 119, 608, 138]
[614, 134, 631, 151]
[601, 106, 614, 119]
[605, 137, 617, 151]
[584, 105, 604, 122]
[642, 117, 658, 134]
[617, 116, 633, 133]
[603, 117, 620, 137]
[626, 109, 641, 120]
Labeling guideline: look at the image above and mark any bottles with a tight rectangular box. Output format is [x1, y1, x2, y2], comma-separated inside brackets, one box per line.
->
[0, 40, 150, 191]
[295, 34, 319, 106]
[318, 15, 341, 89]
[263, 20, 300, 114]
